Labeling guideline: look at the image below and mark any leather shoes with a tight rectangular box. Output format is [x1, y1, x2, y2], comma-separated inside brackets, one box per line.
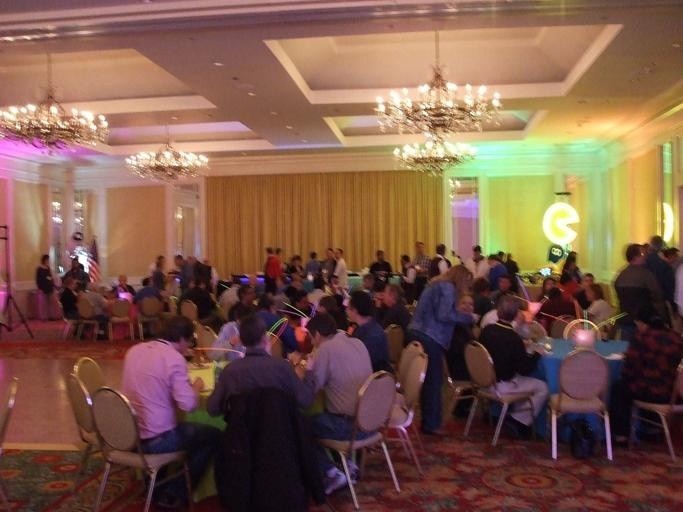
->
[434, 428, 449, 436]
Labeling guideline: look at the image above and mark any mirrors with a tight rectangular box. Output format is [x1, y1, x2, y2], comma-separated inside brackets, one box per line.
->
[166, 189, 206, 265]
[450, 178, 480, 264]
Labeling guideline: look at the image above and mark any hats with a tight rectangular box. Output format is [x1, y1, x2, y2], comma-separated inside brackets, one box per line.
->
[197, 257, 204, 264]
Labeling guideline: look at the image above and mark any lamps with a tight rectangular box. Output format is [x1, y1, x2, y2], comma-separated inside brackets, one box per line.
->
[392, 128, 478, 175]
[125, 125, 210, 186]
[374, 27, 502, 131]
[1, 44, 109, 154]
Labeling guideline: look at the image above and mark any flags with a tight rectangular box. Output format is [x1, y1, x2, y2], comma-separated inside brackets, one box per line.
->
[88, 241, 97, 283]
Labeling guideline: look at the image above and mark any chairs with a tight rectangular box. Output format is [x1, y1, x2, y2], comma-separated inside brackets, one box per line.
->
[627, 359, 683, 462]
[550, 315, 576, 339]
[562, 319, 603, 344]
[314, 370, 401, 508]
[218, 287, 242, 320]
[53, 290, 79, 347]
[265, 330, 284, 359]
[108, 299, 134, 343]
[161, 277, 181, 299]
[195, 326, 218, 348]
[180, 300, 201, 332]
[74, 293, 101, 343]
[1, 378, 19, 509]
[135, 288, 164, 341]
[358, 340, 429, 480]
[67, 373, 142, 478]
[381, 323, 405, 371]
[546, 348, 612, 462]
[439, 352, 495, 433]
[93, 387, 194, 512]
[390, 341, 424, 465]
[164, 296, 179, 321]
[461, 340, 543, 446]
[73, 356, 105, 421]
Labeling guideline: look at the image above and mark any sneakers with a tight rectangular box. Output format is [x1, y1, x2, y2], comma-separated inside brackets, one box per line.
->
[346, 462, 359, 485]
[145, 478, 160, 499]
[160, 493, 180, 507]
[324, 470, 347, 496]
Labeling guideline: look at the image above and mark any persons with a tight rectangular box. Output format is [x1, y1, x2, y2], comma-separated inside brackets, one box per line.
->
[172, 255, 191, 291]
[201, 259, 218, 290]
[325, 247, 336, 282]
[584, 284, 615, 339]
[177, 278, 217, 322]
[264, 247, 272, 272]
[674, 262, 683, 318]
[60, 278, 85, 339]
[151, 256, 166, 290]
[121, 315, 214, 509]
[207, 304, 247, 362]
[537, 278, 554, 302]
[346, 291, 391, 378]
[562, 251, 576, 272]
[397, 255, 417, 304]
[558, 263, 577, 296]
[280, 286, 295, 307]
[567, 256, 580, 282]
[428, 244, 451, 279]
[376, 284, 409, 330]
[228, 284, 256, 319]
[487, 255, 508, 291]
[472, 246, 490, 278]
[402, 265, 480, 438]
[267, 248, 282, 295]
[370, 250, 391, 292]
[576, 273, 593, 309]
[286, 312, 371, 495]
[446, 294, 482, 418]
[207, 314, 314, 419]
[480, 295, 548, 441]
[111, 274, 136, 303]
[480, 290, 524, 333]
[255, 292, 297, 358]
[472, 276, 493, 324]
[36, 254, 59, 321]
[313, 270, 329, 290]
[134, 278, 161, 336]
[412, 242, 431, 301]
[541, 287, 575, 331]
[330, 248, 347, 294]
[373, 279, 385, 316]
[490, 276, 511, 301]
[613, 243, 669, 341]
[606, 300, 682, 450]
[362, 274, 384, 303]
[62, 258, 87, 292]
[283, 289, 318, 321]
[306, 251, 322, 273]
[267, 274, 291, 308]
[645, 235, 674, 302]
[504, 253, 518, 291]
[318, 297, 347, 331]
[663, 247, 680, 262]
[79, 263, 90, 281]
[186, 256, 211, 287]
[498, 251, 505, 263]
[287, 255, 304, 274]
[80, 279, 108, 339]
